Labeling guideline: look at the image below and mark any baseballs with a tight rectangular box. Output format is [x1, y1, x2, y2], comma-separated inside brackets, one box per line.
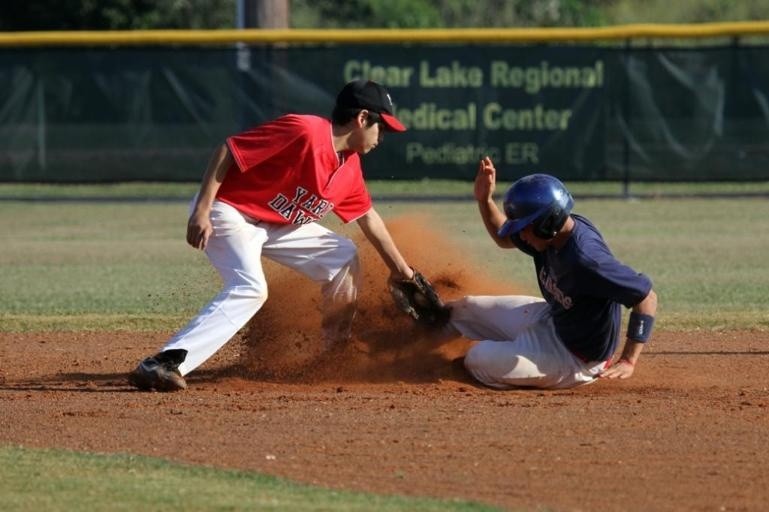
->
[414, 292, 429, 305]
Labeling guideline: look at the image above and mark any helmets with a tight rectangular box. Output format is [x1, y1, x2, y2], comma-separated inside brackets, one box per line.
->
[497, 173, 574, 239]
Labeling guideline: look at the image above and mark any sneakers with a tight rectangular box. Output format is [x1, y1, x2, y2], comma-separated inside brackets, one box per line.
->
[130, 349, 187, 390]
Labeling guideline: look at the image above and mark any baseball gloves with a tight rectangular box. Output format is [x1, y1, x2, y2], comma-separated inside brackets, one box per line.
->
[388, 265, 450, 332]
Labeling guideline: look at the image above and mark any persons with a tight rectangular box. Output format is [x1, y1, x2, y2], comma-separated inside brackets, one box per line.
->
[127, 79, 452, 391]
[351, 156, 658, 390]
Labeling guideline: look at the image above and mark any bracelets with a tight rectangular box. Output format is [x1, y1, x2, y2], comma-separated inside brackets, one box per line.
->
[626, 311, 655, 344]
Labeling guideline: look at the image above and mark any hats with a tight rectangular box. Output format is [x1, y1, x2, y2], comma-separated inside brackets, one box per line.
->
[338, 80, 407, 132]
